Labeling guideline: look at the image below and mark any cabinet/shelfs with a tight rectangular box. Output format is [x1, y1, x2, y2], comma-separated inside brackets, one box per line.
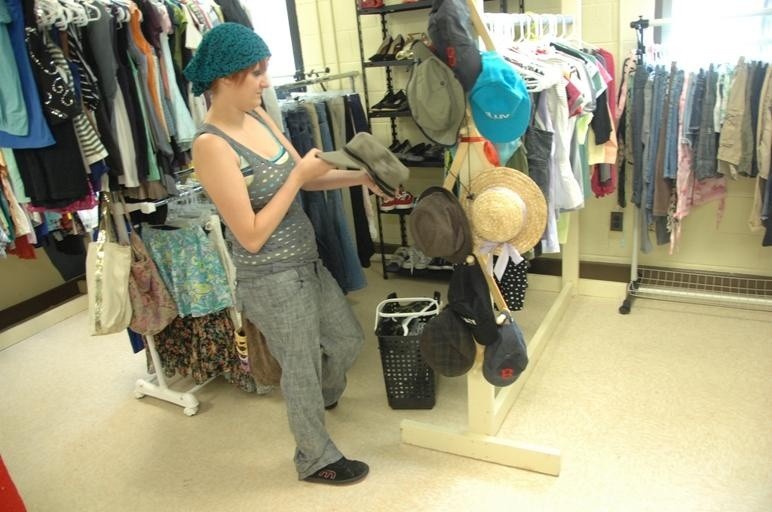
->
[354, 2, 449, 283]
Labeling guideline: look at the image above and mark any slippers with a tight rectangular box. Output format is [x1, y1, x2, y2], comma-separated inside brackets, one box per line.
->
[386, 254, 407, 274]
[395, 243, 408, 259]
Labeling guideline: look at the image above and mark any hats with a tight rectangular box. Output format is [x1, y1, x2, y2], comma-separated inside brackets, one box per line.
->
[481, 308, 530, 387]
[316, 132, 409, 201]
[448, 253, 498, 346]
[409, 185, 473, 265]
[405, 40, 465, 147]
[428, 0, 482, 90]
[420, 307, 475, 377]
[459, 166, 548, 255]
[469, 51, 531, 144]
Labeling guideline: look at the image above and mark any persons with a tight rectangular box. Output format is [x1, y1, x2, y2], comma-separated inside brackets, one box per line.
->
[187, 21, 401, 487]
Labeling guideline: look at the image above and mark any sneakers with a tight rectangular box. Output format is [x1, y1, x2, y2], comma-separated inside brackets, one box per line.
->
[411, 143, 433, 162]
[402, 244, 415, 270]
[389, 140, 399, 151]
[402, 142, 426, 160]
[415, 257, 432, 270]
[394, 139, 412, 155]
[396, 193, 415, 212]
[305, 457, 370, 485]
[380, 201, 394, 212]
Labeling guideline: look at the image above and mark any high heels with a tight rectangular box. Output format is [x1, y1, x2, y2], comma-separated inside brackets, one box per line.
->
[369, 36, 393, 62]
[383, 34, 405, 61]
[370, 93, 395, 112]
[382, 89, 408, 112]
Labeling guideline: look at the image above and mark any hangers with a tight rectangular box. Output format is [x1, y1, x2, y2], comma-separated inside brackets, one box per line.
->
[482, 11, 602, 52]
[149, 184, 218, 229]
[280, 71, 358, 115]
[34, 1, 144, 30]
[623, 44, 772, 73]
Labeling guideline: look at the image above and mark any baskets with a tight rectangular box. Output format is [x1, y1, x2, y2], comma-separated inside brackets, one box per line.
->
[374, 291, 442, 409]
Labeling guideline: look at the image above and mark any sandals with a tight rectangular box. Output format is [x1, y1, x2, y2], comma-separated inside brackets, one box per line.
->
[407, 32, 429, 60]
[395, 32, 417, 61]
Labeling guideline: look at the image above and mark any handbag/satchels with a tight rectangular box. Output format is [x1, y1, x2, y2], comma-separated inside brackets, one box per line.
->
[83, 189, 131, 337]
[111, 191, 180, 337]
